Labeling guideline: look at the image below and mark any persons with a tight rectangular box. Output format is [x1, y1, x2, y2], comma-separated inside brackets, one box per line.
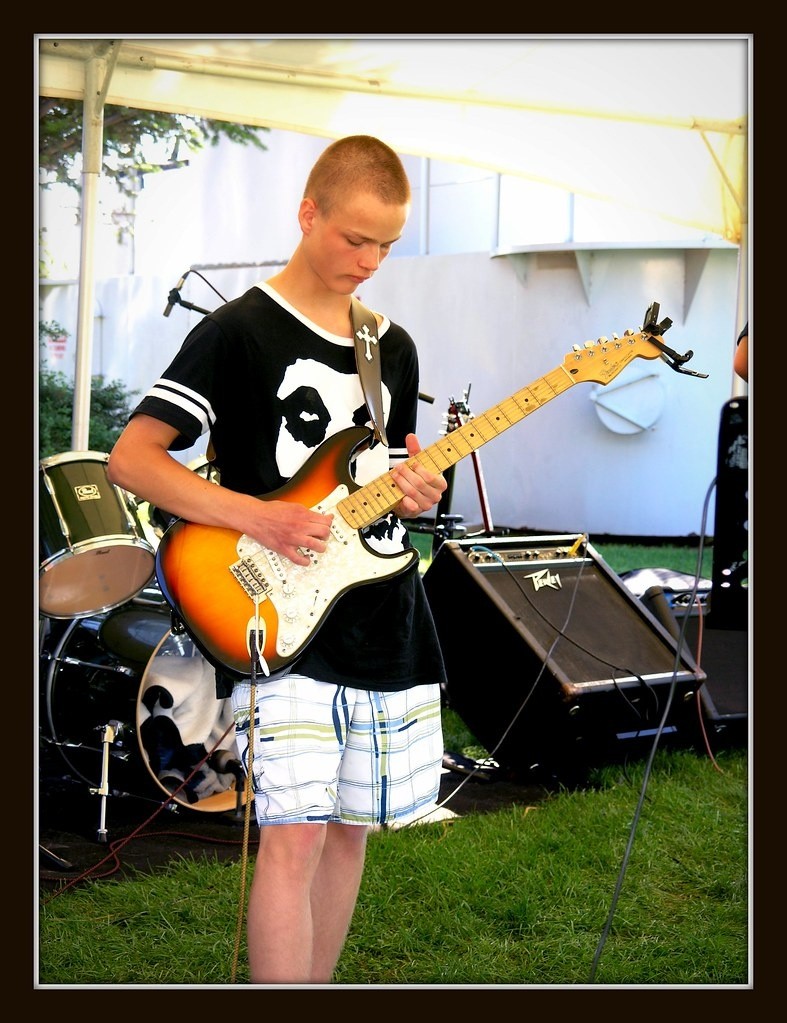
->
[734, 320, 748, 383]
[103, 133, 448, 984]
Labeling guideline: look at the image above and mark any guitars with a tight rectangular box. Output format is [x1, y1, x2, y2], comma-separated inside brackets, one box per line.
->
[153, 301, 690, 685]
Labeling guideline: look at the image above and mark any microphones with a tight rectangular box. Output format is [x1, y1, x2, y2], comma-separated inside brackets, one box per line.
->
[163, 272, 189, 317]
[211, 749, 245, 774]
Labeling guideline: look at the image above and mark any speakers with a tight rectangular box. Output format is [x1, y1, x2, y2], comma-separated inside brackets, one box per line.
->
[423, 534, 707, 794]
[640, 585, 748, 756]
[711, 395, 748, 631]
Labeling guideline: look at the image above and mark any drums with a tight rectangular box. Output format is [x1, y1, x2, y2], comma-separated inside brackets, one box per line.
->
[43, 588, 258, 829]
[146, 455, 224, 542]
[38, 451, 155, 622]
